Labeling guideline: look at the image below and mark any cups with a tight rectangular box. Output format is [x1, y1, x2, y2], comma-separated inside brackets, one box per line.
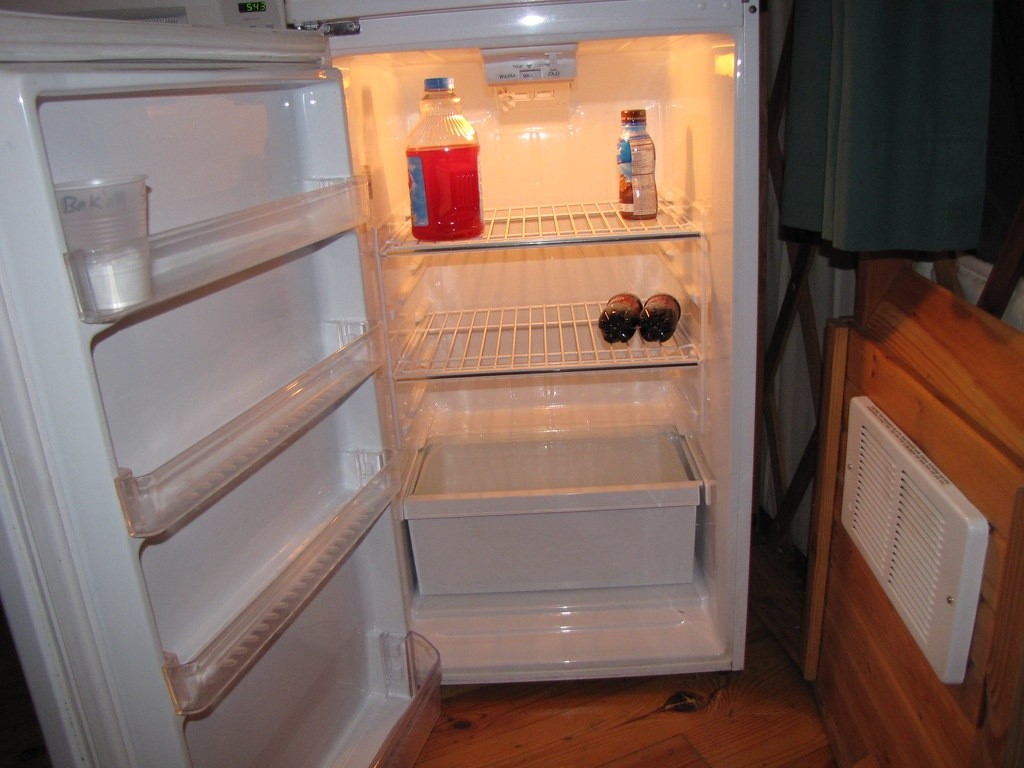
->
[52, 167, 155, 323]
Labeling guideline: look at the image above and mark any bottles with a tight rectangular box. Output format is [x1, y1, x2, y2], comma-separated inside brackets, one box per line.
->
[600, 291, 643, 345]
[618, 108, 658, 220]
[639, 292, 682, 343]
[404, 74, 487, 242]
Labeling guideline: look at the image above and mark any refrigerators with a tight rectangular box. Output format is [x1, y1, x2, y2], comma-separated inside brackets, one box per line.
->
[0, 0, 779, 768]
